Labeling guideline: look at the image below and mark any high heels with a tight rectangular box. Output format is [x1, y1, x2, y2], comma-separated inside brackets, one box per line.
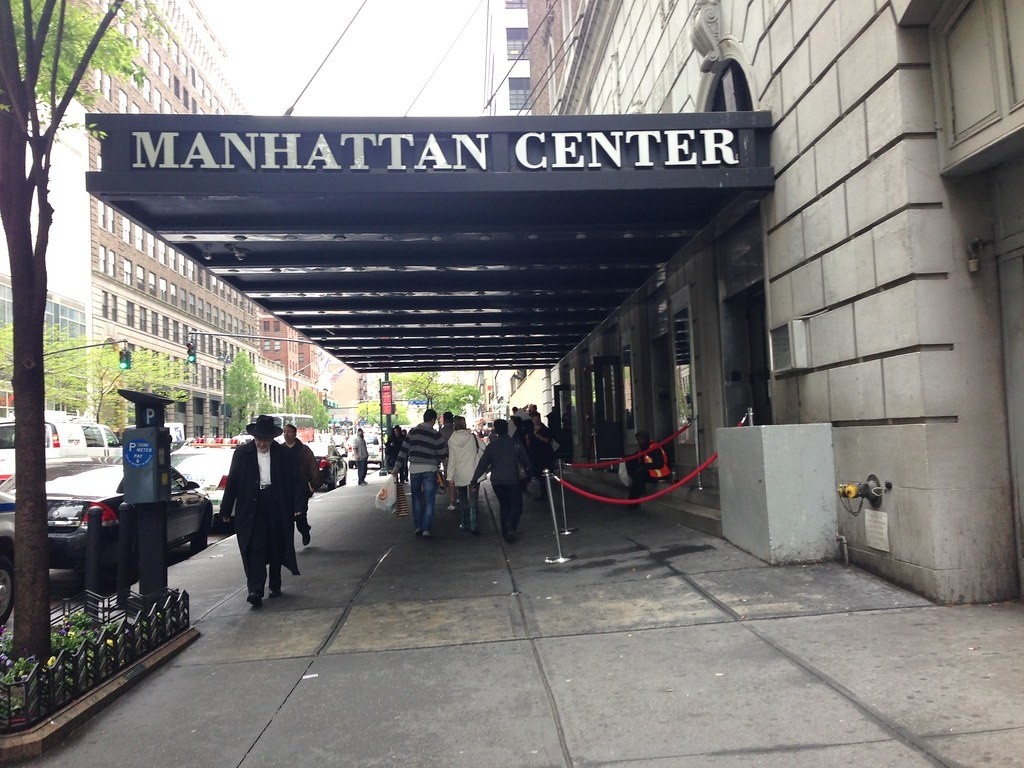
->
[414, 527, 431, 539]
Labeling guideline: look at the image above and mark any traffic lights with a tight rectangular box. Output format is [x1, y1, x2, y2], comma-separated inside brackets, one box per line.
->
[186, 341, 196, 363]
[119, 349, 131, 370]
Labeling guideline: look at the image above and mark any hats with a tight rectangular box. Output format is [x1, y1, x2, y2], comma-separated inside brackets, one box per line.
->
[246, 415, 283, 440]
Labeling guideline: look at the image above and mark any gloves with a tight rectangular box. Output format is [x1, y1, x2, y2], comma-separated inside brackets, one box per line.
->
[471, 480, 479, 489]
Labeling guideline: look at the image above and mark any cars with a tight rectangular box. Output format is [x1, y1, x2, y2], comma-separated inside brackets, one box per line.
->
[168, 423, 384, 531]
[0, 455, 214, 596]
[0, 488, 15, 627]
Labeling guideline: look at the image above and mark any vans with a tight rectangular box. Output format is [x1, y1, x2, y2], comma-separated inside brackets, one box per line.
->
[0, 411, 122, 487]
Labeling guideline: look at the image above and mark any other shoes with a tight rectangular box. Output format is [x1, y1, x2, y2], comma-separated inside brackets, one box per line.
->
[472, 529, 479, 535]
[247, 592, 262, 607]
[269, 587, 281, 597]
[458, 523, 468, 530]
[502, 529, 514, 542]
[302, 530, 310, 545]
[446, 497, 460, 511]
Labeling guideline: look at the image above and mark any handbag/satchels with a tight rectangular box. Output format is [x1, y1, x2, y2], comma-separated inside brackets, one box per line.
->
[472, 432, 491, 470]
[618, 461, 630, 487]
[513, 442, 528, 481]
[395, 477, 409, 518]
[376, 474, 397, 511]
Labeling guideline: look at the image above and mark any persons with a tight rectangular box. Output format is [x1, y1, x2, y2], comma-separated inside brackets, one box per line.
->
[352, 428, 369, 486]
[437, 410, 457, 511]
[390, 409, 448, 536]
[625, 431, 672, 509]
[280, 424, 320, 547]
[218, 416, 306, 607]
[385, 425, 409, 484]
[510, 404, 570, 502]
[469, 419, 524, 543]
[445, 416, 488, 533]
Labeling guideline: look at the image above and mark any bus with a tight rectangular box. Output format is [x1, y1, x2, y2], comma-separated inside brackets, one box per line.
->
[252, 412, 315, 445]
